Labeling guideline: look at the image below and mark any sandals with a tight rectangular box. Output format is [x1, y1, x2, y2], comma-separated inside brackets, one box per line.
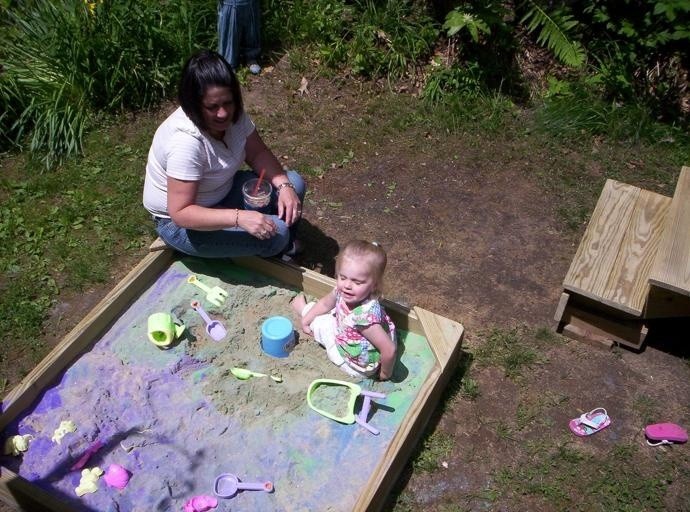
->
[569, 408, 611, 436]
[645, 422, 688, 446]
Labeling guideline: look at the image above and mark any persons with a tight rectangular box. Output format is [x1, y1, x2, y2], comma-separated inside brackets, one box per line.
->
[290, 240, 398, 379]
[142, 48, 307, 260]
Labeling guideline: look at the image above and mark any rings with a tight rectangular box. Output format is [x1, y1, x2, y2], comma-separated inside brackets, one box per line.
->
[298, 210, 302, 213]
[261, 229, 267, 235]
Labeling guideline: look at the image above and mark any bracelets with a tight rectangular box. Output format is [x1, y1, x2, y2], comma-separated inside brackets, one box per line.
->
[235, 207, 238, 229]
[275, 183, 294, 196]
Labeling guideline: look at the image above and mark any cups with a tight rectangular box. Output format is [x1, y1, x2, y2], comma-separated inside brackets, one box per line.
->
[242, 179, 272, 210]
